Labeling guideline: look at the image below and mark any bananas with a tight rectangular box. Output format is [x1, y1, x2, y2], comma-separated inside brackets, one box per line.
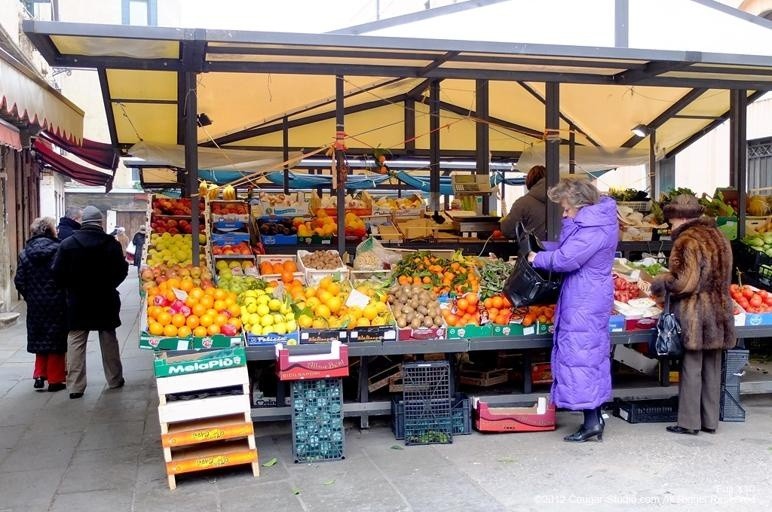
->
[199, 180, 236, 200]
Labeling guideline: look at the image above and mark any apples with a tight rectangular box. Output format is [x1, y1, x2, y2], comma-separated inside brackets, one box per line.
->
[212, 202, 246, 215]
[151, 198, 205, 234]
[613, 277, 640, 304]
[730, 283, 772, 315]
[142, 242, 265, 296]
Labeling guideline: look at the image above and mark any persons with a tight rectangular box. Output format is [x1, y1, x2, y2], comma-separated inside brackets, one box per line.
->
[53, 206, 130, 399]
[501, 162, 547, 251]
[529, 173, 620, 442]
[12, 216, 65, 391]
[112, 225, 145, 267]
[57, 209, 85, 239]
[651, 193, 736, 437]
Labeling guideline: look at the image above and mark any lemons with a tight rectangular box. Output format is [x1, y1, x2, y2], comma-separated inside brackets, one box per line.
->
[238, 287, 297, 335]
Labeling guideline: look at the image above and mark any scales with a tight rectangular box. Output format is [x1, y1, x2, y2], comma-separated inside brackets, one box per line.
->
[452, 215, 502, 238]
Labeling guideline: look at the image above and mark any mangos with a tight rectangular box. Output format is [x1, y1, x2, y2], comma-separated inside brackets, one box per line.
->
[292, 209, 366, 237]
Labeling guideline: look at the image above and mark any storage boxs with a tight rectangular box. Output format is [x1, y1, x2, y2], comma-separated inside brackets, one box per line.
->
[716, 216, 772, 326]
[609, 201, 670, 330]
[138, 188, 556, 343]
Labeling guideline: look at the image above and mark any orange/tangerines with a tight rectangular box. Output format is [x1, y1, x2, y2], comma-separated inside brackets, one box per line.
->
[398, 255, 478, 296]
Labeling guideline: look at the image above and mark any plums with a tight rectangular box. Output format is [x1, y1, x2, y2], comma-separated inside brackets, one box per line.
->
[258, 219, 297, 235]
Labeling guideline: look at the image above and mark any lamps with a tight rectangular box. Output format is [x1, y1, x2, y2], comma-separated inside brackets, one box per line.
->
[196, 112, 212, 127]
[630, 123, 654, 139]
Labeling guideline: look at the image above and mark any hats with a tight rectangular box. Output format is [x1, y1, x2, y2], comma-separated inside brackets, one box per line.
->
[81, 206, 104, 224]
[663, 194, 704, 219]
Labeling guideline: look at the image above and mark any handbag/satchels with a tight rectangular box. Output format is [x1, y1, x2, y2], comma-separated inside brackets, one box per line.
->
[504, 219, 564, 307]
[125, 240, 136, 255]
[652, 273, 688, 358]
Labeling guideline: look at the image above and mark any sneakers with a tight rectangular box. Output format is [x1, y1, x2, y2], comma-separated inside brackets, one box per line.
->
[666, 425, 715, 436]
[33, 376, 84, 400]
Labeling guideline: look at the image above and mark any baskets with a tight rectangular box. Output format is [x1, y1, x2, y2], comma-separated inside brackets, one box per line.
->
[617, 200, 652, 212]
[289, 375, 346, 464]
[612, 393, 681, 424]
[389, 358, 475, 445]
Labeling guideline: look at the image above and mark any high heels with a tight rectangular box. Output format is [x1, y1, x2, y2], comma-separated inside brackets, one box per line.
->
[564, 419, 605, 442]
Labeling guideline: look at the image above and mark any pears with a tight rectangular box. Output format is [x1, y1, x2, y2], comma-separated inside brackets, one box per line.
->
[147, 232, 206, 267]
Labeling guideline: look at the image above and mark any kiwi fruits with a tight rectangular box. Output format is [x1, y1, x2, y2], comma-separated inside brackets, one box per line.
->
[387, 283, 444, 330]
